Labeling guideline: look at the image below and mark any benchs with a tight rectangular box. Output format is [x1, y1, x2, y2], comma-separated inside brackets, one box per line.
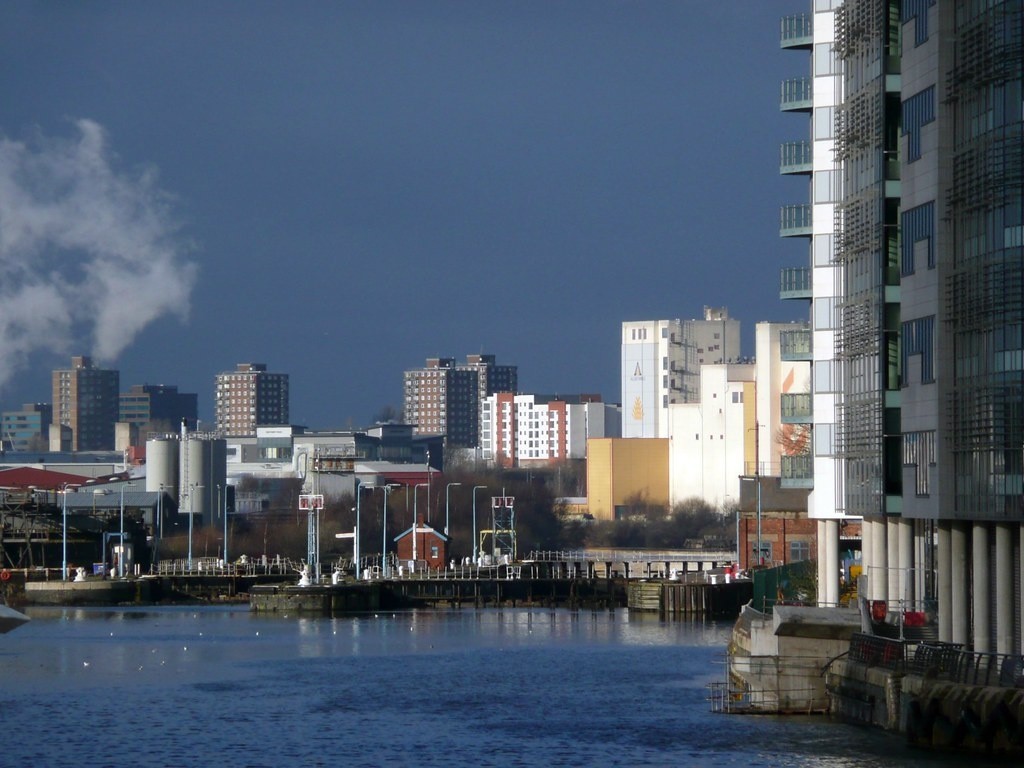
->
[904, 641, 964, 677]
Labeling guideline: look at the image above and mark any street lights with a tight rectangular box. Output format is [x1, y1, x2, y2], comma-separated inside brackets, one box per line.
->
[356, 481, 375, 580]
[412, 483, 429, 563]
[188, 484, 205, 573]
[119, 484, 137, 578]
[472, 485, 488, 564]
[224, 482, 239, 564]
[159, 485, 174, 539]
[445, 482, 462, 536]
[382, 484, 400, 577]
[63, 483, 81, 580]
